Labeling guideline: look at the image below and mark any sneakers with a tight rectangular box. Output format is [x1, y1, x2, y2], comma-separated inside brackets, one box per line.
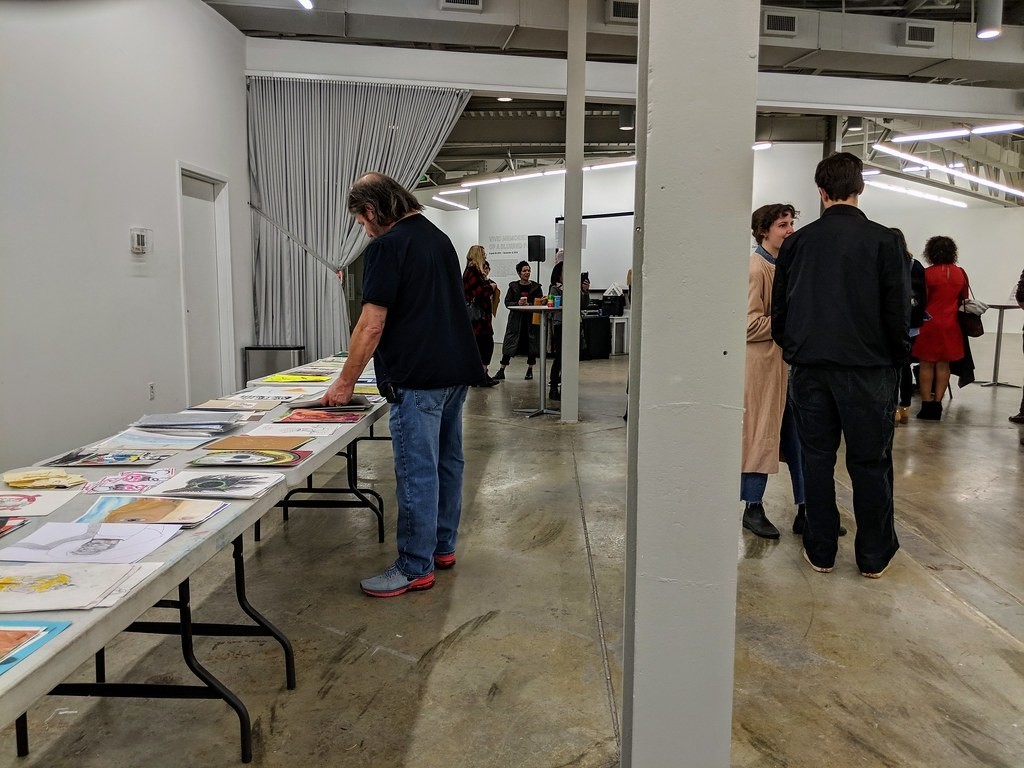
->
[434, 552, 456, 569]
[360, 562, 436, 597]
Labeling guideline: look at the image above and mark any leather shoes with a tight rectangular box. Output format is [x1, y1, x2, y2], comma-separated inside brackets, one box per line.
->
[793, 516, 846, 536]
[743, 503, 780, 539]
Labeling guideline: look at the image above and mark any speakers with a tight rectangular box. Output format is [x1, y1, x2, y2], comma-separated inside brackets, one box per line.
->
[528, 235, 546, 262]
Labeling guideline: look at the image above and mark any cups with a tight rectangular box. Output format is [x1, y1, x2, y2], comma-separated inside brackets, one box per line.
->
[520, 297, 527, 306]
[554, 296, 561, 308]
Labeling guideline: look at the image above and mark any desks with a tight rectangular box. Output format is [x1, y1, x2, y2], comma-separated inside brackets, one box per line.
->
[507, 305, 566, 418]
[606, 316, 629, 358]
[0, 344, 392, 768]
[967, 301, 1022, 389]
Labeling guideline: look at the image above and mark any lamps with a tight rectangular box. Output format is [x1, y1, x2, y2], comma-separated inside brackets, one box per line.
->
[848, 117, 1024, 209]
[617, 104, 634, 131]
[432, 141, 775, 211]
[976, 1, 1003, 39]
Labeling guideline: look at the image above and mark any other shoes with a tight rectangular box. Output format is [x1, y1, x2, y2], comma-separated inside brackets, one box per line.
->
[549, 387, 559, 399]
[1009, 413, 1024, 423]
[802, 546, 833, 573]
[917, 401, 943, 420]
[491, 368, 505, 380]
[525, 368, 533, 380]
[861, 551, 896, 578]
[899, 406, 910, 423]
[480, 370, 499, 387]
[893, 408, 901, 427]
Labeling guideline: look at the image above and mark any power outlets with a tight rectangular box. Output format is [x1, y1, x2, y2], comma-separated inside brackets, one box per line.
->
[148, 381, 154, 401]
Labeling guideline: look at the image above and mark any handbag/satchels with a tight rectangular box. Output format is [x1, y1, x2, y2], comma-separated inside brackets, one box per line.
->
[959, 312, 984, 337]
[466, 305, 486, 322]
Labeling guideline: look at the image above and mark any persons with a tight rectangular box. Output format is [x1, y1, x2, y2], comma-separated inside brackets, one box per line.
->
[321, 172, 485, 596]
[1007, 269, 1024, 425]
[912, 236, 970, 422]
[492, 260, 544, 380]
[462, 244, 500, 388]
[890, 225, 926, 426]
[770, 152, 914, 578]
[548, 260, 591, 402]
[740, 202, 849, 538]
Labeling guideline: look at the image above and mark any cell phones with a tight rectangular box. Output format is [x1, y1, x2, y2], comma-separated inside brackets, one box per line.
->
[581, 272, 588, 291]
